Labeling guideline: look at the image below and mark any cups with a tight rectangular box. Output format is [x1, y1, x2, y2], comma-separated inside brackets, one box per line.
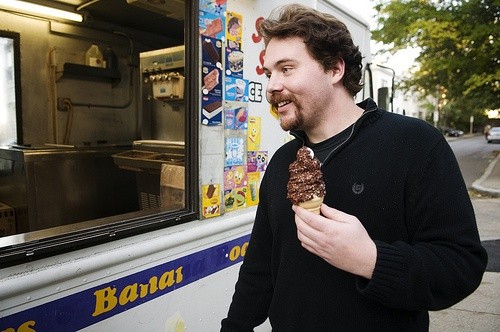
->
[250, 182, 256, 201]
[236, 78, 246, 101]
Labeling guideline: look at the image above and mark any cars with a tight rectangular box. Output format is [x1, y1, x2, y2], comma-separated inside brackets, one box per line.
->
[486, 126, 500, 143]
[447, 129, 464, 137]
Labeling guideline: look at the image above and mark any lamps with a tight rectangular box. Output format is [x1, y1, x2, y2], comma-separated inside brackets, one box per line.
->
[0, 0, 84, 23]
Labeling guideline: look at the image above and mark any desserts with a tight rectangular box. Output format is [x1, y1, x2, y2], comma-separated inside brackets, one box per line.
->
[202, 101, 222, 120]
[228, 50, 243, 72]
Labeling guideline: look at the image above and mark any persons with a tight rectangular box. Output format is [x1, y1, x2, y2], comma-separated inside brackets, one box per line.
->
[219, 3, 489, 332]
[484, 122, 492, 141]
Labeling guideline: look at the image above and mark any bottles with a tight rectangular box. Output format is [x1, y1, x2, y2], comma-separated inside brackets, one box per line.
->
[85, 42, 118, 69]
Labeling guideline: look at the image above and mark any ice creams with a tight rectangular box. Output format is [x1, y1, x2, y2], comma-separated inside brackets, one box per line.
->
[286, 146, 326, 215]
